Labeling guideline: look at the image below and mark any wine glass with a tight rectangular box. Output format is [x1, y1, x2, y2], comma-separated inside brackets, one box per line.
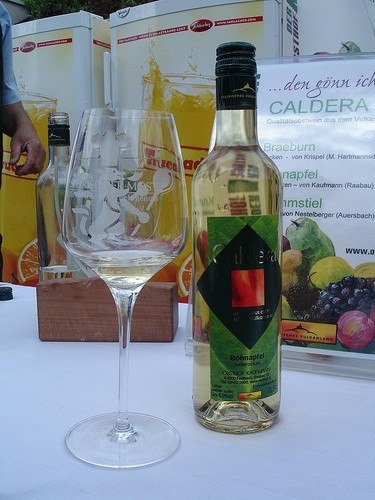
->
[54, 106, 192, 469]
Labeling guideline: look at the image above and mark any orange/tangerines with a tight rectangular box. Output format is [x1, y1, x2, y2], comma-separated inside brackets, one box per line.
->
[147, 253, 193, 297]
[1, 238, 39, 283]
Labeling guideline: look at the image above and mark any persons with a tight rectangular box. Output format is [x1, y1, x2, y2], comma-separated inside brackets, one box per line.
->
[0, 2, 46, 282]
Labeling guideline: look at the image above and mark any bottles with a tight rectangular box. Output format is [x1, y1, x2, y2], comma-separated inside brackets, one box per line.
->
[35, 112, 75, 281]
[187, 42, 284, 433]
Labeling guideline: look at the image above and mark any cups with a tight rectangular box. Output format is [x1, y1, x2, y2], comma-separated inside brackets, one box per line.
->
[0, 91, 57, 285]
[139, 73, 217, 272]
